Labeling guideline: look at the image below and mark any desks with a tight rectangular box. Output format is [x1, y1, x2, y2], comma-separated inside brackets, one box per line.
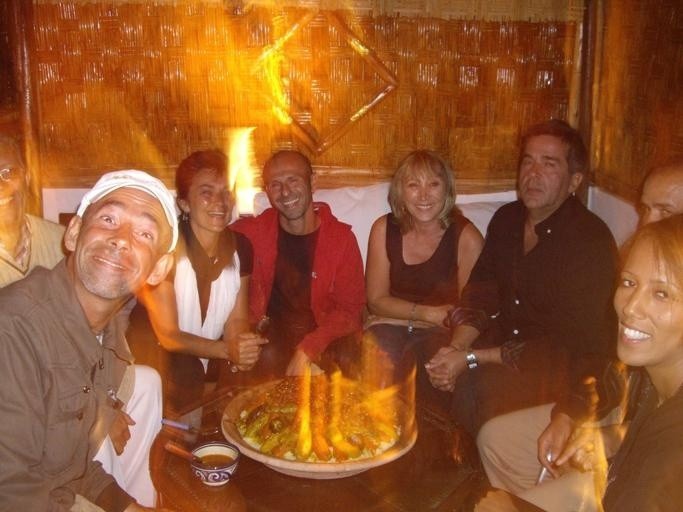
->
[150, 362, 489, 511]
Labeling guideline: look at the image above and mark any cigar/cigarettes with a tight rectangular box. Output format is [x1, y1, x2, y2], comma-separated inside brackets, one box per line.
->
[537, 448, 553, 485]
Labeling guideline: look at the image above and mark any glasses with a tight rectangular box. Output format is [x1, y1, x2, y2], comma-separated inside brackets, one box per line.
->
[0, 167, 21, 182]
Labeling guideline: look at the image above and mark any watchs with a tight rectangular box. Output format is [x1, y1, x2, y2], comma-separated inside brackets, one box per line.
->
[226, 361, 237, 374]
[465, 347, 478, 370]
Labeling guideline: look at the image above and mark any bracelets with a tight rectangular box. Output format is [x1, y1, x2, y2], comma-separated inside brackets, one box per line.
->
[407, 320, 413, 333]
[407, 303, 417, 317]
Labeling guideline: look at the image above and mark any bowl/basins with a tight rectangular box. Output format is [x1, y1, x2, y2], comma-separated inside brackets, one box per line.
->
[220, 378, 422, 481]
[188, 443, 239, 488]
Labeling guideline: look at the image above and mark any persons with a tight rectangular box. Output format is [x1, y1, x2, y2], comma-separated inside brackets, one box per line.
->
[415, 118, 624, 430]
[365, 149, 485, 412]
[1, 168, 180, 511]
[474, 213, 683, 512]
[1, 133, 164, 512]
[125, 149, 269, 410]
[226, 151, 368, 378]
[475, 160, 682, 512]
